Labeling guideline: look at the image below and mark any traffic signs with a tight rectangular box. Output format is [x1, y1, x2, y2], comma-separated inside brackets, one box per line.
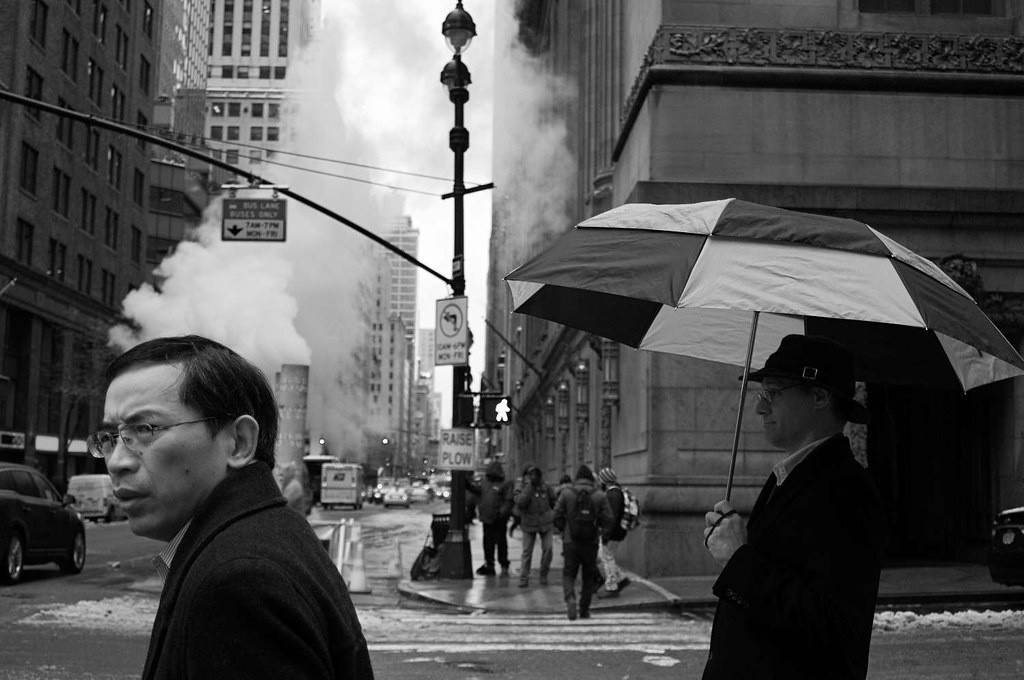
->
[222, 198, 288, 242]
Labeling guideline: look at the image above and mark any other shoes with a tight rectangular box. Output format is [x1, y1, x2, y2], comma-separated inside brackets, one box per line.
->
[567, 598, 577, 620]
[501, 566, 508, 573]
[539, 574, 548, 584]
[598, 589, 619, 601]
[618, 578, 630, 591]
[476, 565, 497, 575]
[579, 607, 589, 618]
[518, 580, 527, 587]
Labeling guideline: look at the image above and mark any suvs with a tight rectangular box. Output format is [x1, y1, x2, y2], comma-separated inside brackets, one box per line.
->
[1, 463, 86, 587]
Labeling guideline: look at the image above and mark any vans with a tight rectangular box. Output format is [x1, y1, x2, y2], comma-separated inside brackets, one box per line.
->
[66, 474, 129, 523]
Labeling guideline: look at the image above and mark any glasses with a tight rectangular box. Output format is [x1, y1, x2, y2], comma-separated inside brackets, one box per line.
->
[86, 415, 221, 459]
[756, 381, 806, 404]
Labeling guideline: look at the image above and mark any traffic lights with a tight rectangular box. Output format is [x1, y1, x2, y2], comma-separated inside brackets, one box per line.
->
[381, 437, 389, 445]
[319, 438, 326, 445]
[479, 394, 512, 424]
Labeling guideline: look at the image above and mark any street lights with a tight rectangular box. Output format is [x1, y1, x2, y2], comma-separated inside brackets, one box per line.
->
[440, 1, 477, 580]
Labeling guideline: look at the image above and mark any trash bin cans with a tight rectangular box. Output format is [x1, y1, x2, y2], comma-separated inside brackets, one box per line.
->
[431, 514, 469, 548]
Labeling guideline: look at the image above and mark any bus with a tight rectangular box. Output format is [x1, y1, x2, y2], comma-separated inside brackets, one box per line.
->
[303, 455, 365, 511]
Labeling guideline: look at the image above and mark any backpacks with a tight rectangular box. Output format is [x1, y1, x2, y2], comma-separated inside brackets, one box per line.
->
[567, 486, 600, 540]
[607, 485, 639, 530]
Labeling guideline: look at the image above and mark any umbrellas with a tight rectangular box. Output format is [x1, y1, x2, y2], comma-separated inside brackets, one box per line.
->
[501, 198, 1024, 501]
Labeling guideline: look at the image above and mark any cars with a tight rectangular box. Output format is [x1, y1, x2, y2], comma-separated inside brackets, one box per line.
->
[361, 476, 450, 507]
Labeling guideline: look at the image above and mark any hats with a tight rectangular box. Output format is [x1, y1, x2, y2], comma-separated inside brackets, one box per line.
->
[599, 468, 616, 484]
[738, 333, 870, 425]
[576, 465, 595, 481]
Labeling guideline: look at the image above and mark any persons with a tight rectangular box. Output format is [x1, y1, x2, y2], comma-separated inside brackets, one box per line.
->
[279, 462, 306, 518]
[467, 461, 630, 621]
[704, 335, 882, 680]
[87, 335, 377, 680]
